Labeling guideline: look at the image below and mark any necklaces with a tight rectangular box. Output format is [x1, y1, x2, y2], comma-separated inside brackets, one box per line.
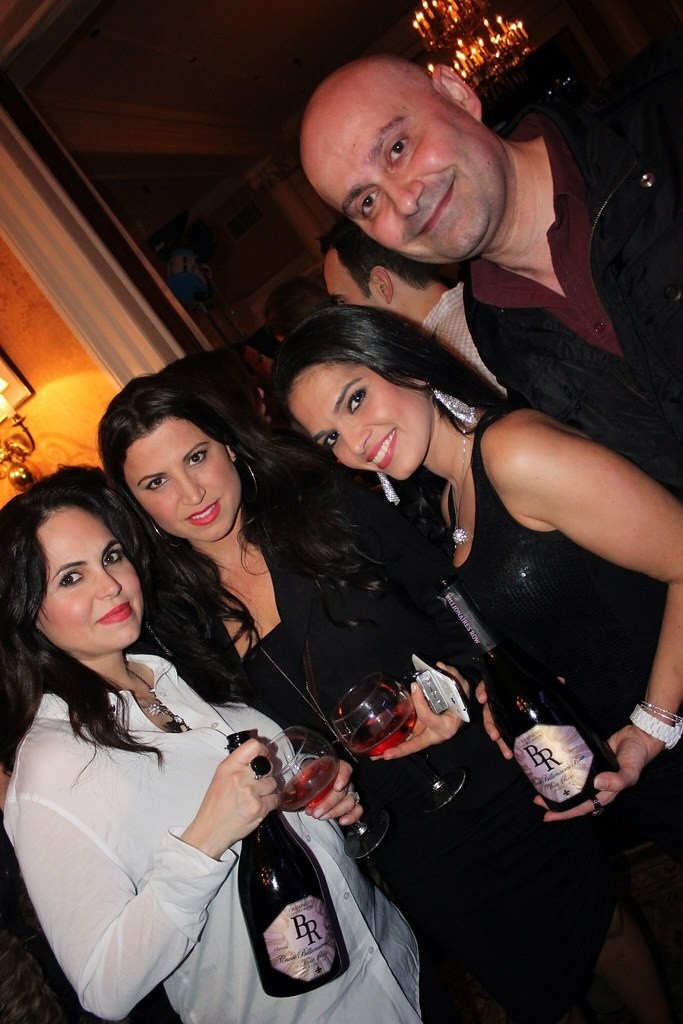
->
[123, 667, 192, 735]
[450, 429, 469, 545]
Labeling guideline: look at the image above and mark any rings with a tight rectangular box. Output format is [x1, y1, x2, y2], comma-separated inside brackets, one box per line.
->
[247, 755, 273, 780]
[347, 789, 362, 805]
[591, 795, 606, 819]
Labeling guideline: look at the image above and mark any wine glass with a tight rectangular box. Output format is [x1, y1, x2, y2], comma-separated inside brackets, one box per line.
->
[261, 726, 390, 859]
[330, 671, 466, 814]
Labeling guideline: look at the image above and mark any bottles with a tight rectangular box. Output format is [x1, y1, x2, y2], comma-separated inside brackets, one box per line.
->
[226, 728, 349, 998]
[436, 573, 620, 812]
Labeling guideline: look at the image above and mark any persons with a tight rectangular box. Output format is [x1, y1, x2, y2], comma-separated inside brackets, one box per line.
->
[299, 27, 683, 497]
[100, 370, 675, 1024]
[317, 205, 508, 401]
[2, 472, 455, 1024]
[154, 279, 316, 445]
[272, 299, 683, 865]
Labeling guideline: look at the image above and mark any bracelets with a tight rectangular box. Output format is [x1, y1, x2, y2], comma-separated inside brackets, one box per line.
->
[638, 698, 683, 725]
[630, 703, 683, 751]
